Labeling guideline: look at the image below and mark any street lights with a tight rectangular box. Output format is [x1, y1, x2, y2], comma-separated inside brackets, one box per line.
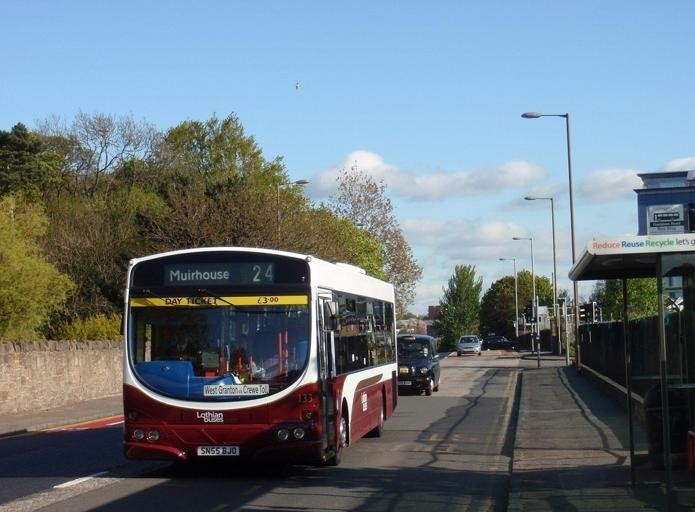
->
[273, 178, 310, 250]
[520, 109, 583, 369]
[496, 194, 559, 340]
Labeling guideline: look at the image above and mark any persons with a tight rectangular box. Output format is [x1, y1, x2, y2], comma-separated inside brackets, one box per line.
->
[397, 344, 404, 356]
[164, 328, 200, 360]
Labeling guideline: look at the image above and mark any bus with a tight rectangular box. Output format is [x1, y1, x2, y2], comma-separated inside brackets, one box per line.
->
[121, 248, 400, 466]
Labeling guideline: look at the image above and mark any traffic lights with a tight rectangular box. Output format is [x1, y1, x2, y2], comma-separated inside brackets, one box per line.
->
[580, 304, 587, 322]
[524, 309, 528, 320]
[526, 300, 532, 316]
[587, 302, 594, 323]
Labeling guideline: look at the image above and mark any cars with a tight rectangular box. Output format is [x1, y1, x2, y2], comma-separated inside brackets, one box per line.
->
[455, 334, 519, 355]
[396, 333, 440, 396]
